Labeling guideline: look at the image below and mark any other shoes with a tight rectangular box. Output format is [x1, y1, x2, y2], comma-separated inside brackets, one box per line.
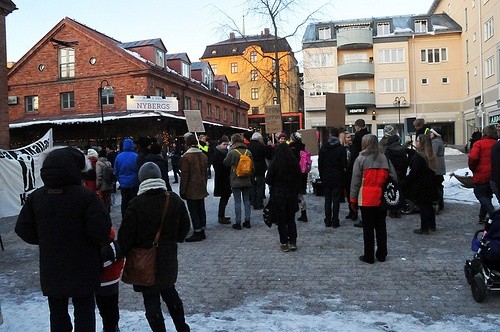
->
[243, 221, 250, 228]
[359, 256, 370, 263]
[185, 231, 205, 242]
[323, 218, 331, 227]
[332, 219, 339, 227]
[478, 218, 486, 223]
[346, 214, 351, 219]
[414, 229, 429, 235]
[429, 226, 436, 230]
[289, 243, 297, 250]
[351, 213, 357, 220]
[280, 243, 290, 251]
[232, 223, 241, 230]
[354, 221, 363, 227]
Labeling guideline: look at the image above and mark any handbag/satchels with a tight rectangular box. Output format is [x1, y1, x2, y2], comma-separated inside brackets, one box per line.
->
[383, 174, 405, 210]
[121, 247, 157, 287]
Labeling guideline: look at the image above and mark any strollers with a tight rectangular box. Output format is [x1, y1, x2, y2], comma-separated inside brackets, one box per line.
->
[463, 209, 500, 303]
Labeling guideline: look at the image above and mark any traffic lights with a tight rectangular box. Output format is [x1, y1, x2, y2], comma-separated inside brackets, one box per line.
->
[372, 111, 376, 115]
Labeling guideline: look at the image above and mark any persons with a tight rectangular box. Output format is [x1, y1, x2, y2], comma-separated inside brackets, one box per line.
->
[379, 124, 413, 217]
[16, 147, 124, 332]
[117, 163, 191, 332]
[338, 118, 369, 226]
[288, 132, 308, 222]
[85, 138, 171, 219]
[350, 134, 389, 263]
[319, 128, 347, 228]
[169, 131, 213, 241]
[211, 131, 288, 229]
[467, 119, 500, 223]
[266, 143, 302, 252]
[409, 118, 444, 235]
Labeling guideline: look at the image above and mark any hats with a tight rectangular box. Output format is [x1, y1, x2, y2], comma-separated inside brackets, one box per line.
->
[87, 148, 97, 156]
[139, 162, 162, 181]
[291, 132, 302, 142]
[278, 134, 288, 140]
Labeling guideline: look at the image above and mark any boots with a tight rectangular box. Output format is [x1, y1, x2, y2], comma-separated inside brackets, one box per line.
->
[218, 214, 231, 224]
[298, 210, 307, 222]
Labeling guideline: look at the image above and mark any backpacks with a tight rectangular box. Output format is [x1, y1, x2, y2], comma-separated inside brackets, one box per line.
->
[292, 147, 311, 173]
[233, 148, 252, 177]
[100, 161, 115, 183]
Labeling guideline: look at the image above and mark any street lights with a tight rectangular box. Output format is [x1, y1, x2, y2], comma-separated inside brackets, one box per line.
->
[98, 79, 115, 155]
[393, 96, 407, 143]
